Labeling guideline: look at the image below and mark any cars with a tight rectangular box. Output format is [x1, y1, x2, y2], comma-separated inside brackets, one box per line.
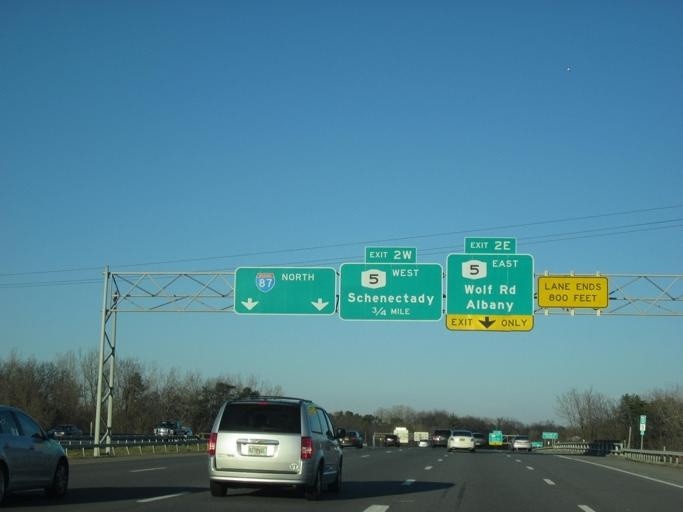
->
[339, 431, 364, 448]
[383, 428, 532, 452]
[47, 424, 83, 438]
[0, 405, 69, 507]
[154, 421, 192, 436]
[209, 396, 344, 497]
